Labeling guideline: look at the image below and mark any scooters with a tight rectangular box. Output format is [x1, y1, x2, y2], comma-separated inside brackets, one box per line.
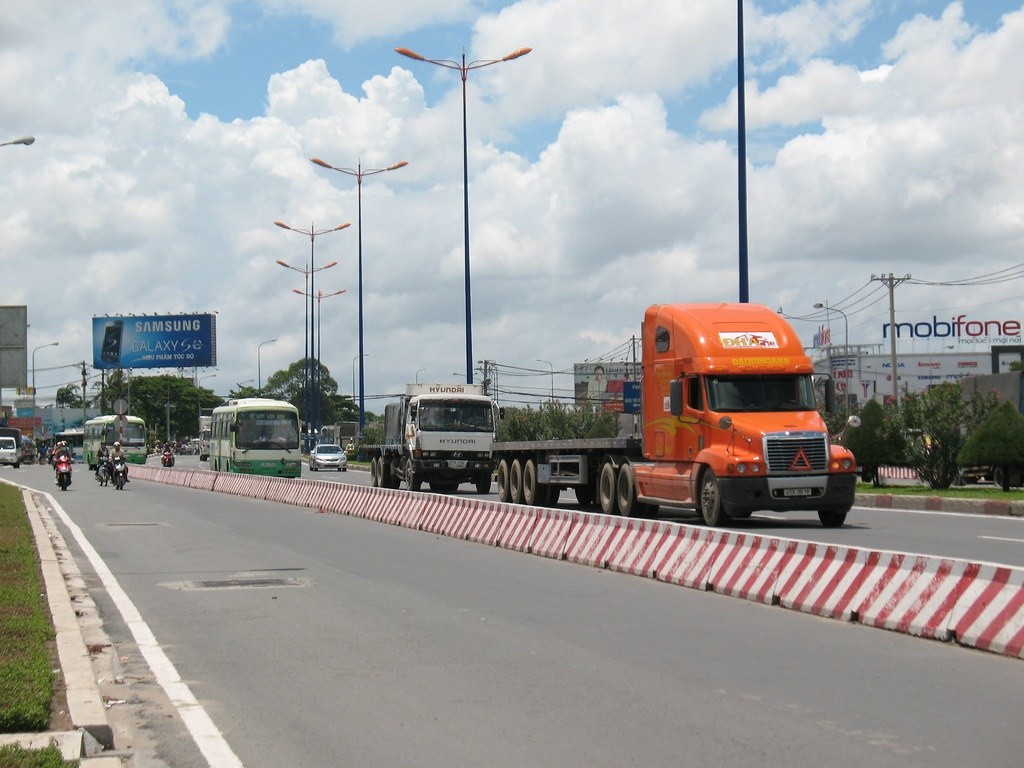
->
[161, 451, 175, 468]
[95, 455, 127, 490]
[54, 453, 72, 491]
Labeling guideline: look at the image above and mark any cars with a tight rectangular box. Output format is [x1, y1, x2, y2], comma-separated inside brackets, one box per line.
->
[308, 444, 348, 471]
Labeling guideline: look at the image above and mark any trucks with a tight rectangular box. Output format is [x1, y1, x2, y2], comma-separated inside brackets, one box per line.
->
[950, 370, 1024, 488]
[0, 427, 26, 469]
[199, 416, 213, 461]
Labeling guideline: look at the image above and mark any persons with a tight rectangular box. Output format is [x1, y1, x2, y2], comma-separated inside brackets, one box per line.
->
[587, 366, 607, 399]
[164, 442, 170, 453]
[444, 409, 451, 419]
[769, 386, 780, 405]
[21, 440, 73, 487]
[261, 425, 269, 441]
[467, 409, 483, 426]
[96, 439, 130, 484]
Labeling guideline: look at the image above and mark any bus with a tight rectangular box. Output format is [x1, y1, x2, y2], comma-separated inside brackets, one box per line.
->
[82, 415, 147, 470]
[209, 397, 308, 479]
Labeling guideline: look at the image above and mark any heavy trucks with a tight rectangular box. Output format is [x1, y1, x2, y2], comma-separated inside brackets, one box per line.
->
[357, 385, 505, 495]
[489, 302, 861, 528]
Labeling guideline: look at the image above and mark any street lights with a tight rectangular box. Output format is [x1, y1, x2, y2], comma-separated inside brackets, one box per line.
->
[812, 304, 848, 425]
[274, 219, 351, 452]
[394, 46, 534, 384]
[276, 259, 346, 454]
[199, 374, 216, 417]
[311, 157, 409, 432]
[536, 360, 553, 401]
[353, 354, 370, 404]
[258, 339, 277, 398]
[32, 342, 60, 442]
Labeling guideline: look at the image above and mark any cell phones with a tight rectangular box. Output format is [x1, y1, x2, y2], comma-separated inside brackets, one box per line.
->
[101, 320, 123, 363]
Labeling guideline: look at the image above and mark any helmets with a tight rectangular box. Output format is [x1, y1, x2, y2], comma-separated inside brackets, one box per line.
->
[114, 442, 120, 447]
[165, 443, 169, 446]
[60, 440, 67, 446]
[101, 441, 106, 444]
